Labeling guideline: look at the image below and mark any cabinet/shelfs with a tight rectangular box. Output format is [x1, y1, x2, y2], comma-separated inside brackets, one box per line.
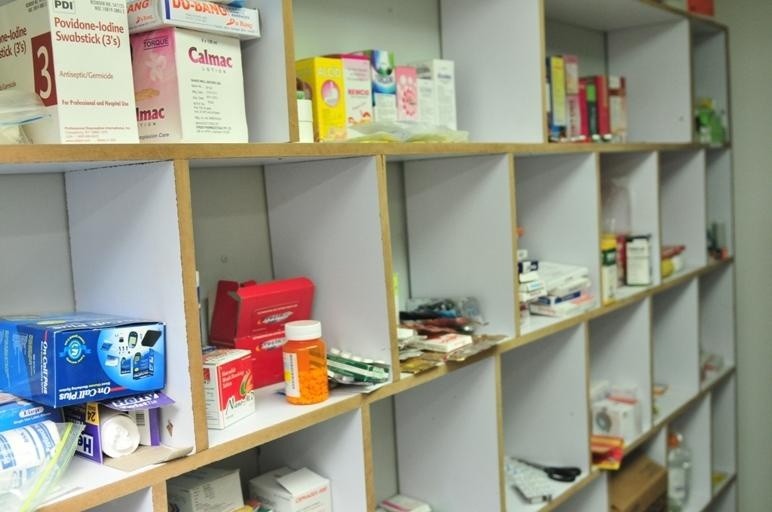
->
[0, 0, 739, 511]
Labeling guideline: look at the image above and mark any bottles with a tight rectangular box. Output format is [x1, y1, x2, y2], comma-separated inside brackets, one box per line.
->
[281, 318, 329, 404]
[669, 434, 692, 504]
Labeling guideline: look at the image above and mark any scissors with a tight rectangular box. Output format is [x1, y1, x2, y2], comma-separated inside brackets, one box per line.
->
[521, 460, 582, 482]
